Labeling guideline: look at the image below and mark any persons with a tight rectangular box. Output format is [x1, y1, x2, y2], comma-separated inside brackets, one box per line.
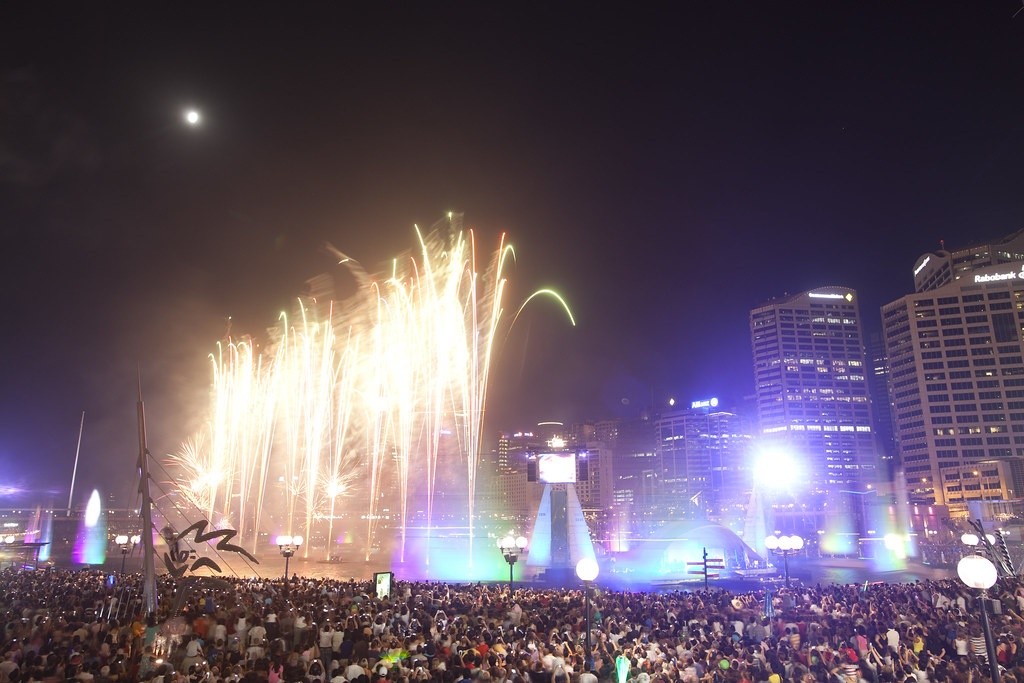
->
[332, 626, 344, 652]
[400, 603, 409, 626]
[729, 614, 744, 636]
[0, 564, 1024, 683]
[373, 613, 386, 636]
[247, 621, 267, 646]
[875, 625, 888, 655]
[969, 624, 988, 662]
[73, 622, 88, 642]
[688, 613, 699, 629]
[608, 620, 623, 650]
[185, 635, 204, 656]
[215, 618, 227, 644]
[345, 655, 365, 681]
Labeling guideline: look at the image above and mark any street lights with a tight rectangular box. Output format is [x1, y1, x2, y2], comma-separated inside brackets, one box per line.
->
[764, 533, 805, 588]
[575, 558, 599, 672]
[957, 555, 998, 683]
[959, 533, 981, 559]
[978, 534, 996, 555]
[276, 535, 304, 588]
[496, 535, 528, 591]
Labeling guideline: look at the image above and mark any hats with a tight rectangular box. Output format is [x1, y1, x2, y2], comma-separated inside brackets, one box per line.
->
[378, 667, 387, 676]
[71, 655, 82, 665]
